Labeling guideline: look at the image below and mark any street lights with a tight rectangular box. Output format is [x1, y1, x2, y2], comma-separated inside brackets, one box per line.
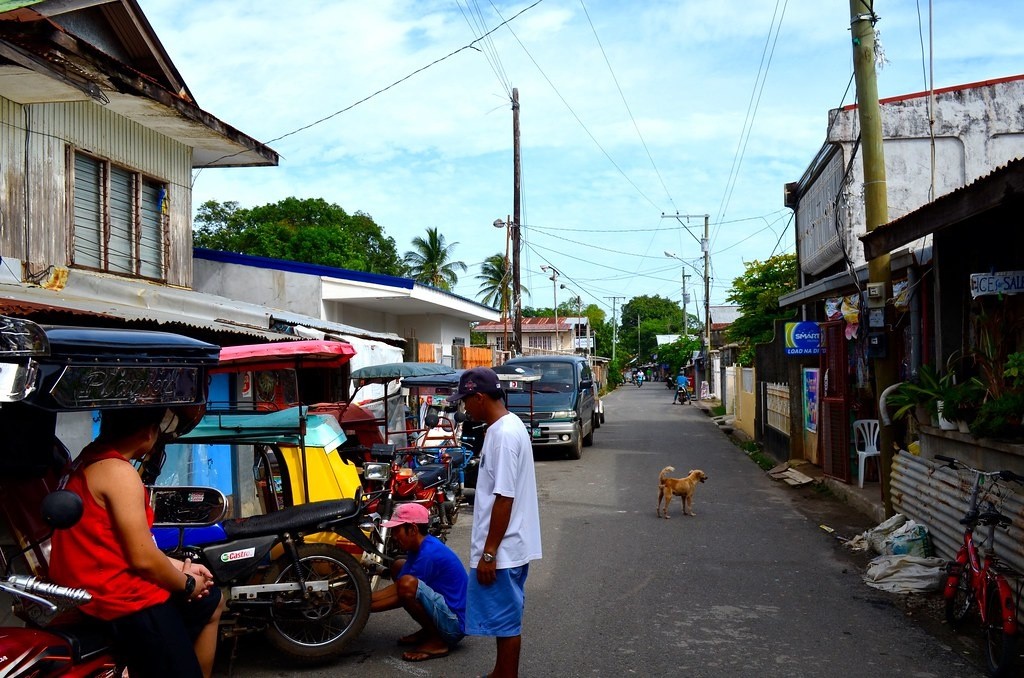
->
[493, 218, 522, 354]
[540, 265, 559, 350]
[663, 249, 712, 393]
[559, 284, 581, 354]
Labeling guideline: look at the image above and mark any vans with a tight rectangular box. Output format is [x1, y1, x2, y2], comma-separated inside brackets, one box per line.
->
[499, 355, 605, 460]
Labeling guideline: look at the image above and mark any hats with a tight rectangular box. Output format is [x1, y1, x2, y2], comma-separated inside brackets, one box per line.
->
[379, 503, 429, 527]
[445, 366, 501, 401]
[680, 371, 685, 375]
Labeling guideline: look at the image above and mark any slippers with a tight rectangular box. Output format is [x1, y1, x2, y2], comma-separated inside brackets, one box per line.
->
[397, 633, 423, 646]
[402, 646, 449, 662]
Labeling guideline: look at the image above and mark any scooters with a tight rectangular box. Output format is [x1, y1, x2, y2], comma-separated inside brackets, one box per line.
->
[636, 374, 642, 387]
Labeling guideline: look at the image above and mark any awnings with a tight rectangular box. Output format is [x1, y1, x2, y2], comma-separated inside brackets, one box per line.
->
[1, 283, 409, 343]
[778, 248, 914, 310]
[855, 154, 1024, 261]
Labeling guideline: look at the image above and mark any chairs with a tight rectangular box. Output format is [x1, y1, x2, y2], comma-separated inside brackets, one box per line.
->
[853, 419, 881, 489]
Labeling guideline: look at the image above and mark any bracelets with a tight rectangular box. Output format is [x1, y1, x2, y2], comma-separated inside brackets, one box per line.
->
[183, 573, 196, 596]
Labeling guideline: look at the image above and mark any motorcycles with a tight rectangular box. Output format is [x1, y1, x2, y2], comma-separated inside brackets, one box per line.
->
[677, 385, 688, 405]
[0, 311, 543, 678]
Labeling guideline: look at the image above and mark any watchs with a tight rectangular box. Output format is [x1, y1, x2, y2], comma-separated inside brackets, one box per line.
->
[482, 553, 496, 563]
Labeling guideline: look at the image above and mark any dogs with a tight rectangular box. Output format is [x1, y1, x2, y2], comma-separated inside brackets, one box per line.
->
[656, 467, 709, 519]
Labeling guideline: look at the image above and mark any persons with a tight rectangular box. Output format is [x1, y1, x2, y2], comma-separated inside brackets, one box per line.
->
[446, 366, 542, 678]
[50, 406, 224, 678]
[334, 502, 469, 662]
[636, 369, 644, 383]
[672, 371, 691, 404]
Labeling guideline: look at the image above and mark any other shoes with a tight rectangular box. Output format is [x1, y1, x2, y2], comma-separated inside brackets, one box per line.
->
[672, 402, 676, 404]
[689, 401, 691, 405]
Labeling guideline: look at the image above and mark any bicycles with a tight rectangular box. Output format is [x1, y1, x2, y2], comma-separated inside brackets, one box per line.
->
[933, 454, 1024, 677]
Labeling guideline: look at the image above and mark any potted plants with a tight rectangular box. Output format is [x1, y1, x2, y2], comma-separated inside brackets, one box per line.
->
[885, 349, 1024, 442]
